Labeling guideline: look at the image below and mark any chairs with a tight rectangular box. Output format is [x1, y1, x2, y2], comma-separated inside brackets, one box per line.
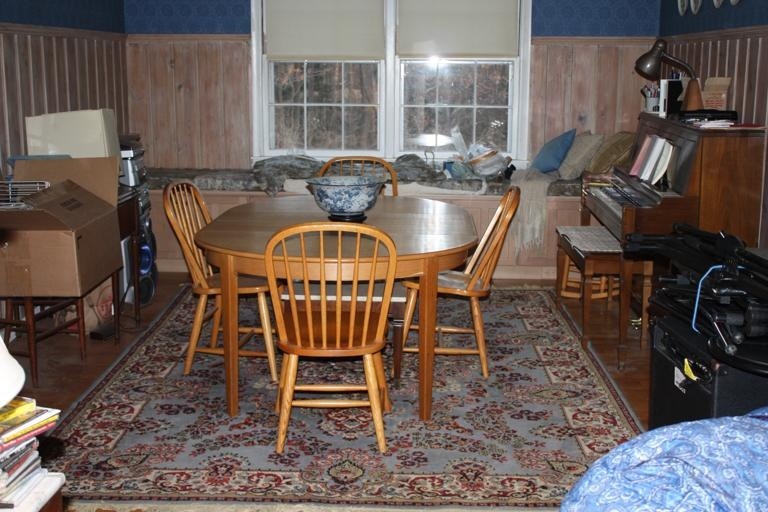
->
[161, 176, 288, 382]
[263, 220, 400, 455]
[315, 155, 398, 196]
[388, 183, 521, 380]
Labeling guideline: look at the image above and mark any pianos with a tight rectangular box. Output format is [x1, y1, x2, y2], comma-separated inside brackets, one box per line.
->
[581, 111, 768, 370]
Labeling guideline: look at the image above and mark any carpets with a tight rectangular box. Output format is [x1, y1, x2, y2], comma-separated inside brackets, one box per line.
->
[38, 280, 648, 511]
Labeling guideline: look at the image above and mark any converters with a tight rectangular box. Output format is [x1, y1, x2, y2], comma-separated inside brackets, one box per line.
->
[90, 326, 114, 339]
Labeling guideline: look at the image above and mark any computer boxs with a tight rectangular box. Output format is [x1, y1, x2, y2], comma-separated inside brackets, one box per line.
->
[25, 107, 125, 190]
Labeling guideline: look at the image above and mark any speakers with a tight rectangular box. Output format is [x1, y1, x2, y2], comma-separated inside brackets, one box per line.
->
[119, 235, 155, 308]
[646, 305, 768, 431]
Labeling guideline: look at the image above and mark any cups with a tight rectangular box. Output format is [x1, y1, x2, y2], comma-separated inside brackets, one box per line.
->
[645, 98, 658, 112]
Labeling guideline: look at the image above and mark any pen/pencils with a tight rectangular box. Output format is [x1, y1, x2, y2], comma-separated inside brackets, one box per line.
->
[671, 66, 688, 80]
[640, 80, 660, 112]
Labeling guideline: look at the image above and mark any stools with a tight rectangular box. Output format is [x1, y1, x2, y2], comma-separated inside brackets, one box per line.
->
[1, 291, 89, 388]
[553, 225, 654, 351]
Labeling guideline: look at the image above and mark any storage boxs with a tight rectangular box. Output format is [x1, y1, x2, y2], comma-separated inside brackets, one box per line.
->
[0, 155, 124, 299]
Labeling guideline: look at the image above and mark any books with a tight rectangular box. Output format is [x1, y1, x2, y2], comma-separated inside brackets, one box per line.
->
[628, 132, 674, 187]
[0, 392, 70, 512]
[677, 107, 767, 133]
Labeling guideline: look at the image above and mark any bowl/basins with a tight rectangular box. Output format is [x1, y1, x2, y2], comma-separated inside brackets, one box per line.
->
[306, 177, 387, 215]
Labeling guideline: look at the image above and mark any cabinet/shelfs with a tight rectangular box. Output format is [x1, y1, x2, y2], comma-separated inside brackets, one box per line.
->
[103, 188, 145, 340]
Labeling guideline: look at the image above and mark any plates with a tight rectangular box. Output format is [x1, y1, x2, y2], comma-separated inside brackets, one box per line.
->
[677, 1, 741, 17]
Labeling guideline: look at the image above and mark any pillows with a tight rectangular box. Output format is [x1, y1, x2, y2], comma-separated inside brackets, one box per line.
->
[558, 128, 606, 180]
[584, 130, 638, 175]
[530, 129, 577, 173]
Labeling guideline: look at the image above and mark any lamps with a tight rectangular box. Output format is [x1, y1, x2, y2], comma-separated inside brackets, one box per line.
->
[634, 39, 705, 111]
